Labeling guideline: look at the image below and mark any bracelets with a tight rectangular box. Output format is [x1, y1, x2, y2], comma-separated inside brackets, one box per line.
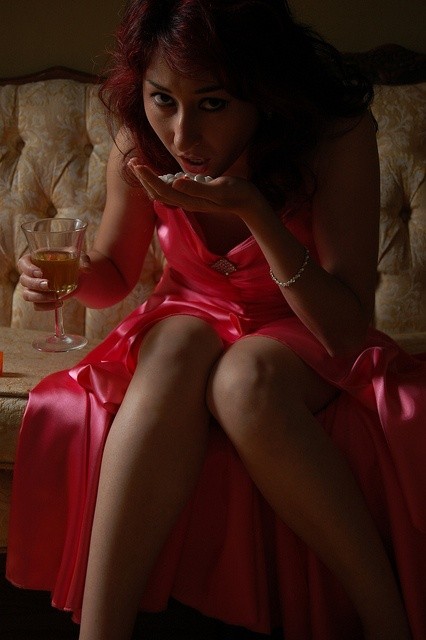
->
[268, 246, 310, 288]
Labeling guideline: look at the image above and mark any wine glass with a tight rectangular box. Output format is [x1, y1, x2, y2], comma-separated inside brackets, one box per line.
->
[21, 218, 89, 353]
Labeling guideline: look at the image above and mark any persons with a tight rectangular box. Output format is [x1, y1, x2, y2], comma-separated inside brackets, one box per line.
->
[5, 0, 426, 640]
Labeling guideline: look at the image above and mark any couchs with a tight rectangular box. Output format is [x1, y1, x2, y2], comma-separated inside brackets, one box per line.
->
[0, 42, 426, 554]
